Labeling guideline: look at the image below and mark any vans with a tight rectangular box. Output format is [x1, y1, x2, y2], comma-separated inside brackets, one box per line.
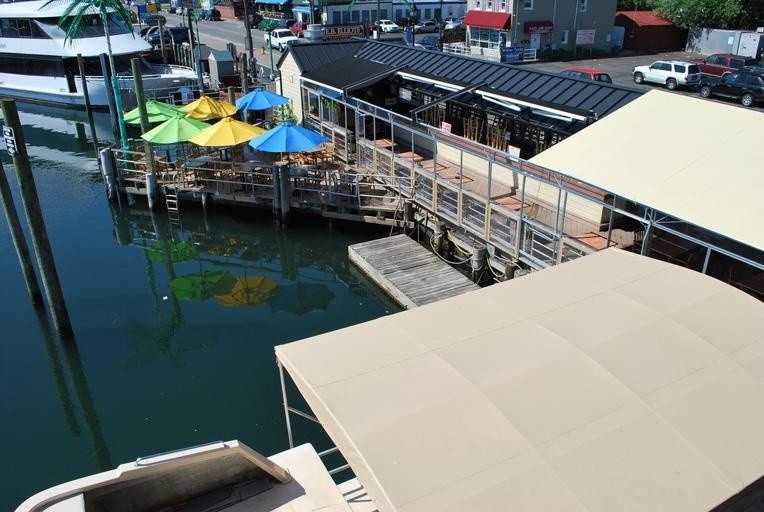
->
[689, 53, 759, 79]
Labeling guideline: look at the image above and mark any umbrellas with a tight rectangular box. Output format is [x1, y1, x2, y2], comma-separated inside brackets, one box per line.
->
[145, 236, 198, 263]
[140, 110, 211, 159]
[189, 116, 266, 161]
[215, 265, 280, 306]
[249, 120, 325, 164]
[264, 267, 336, 315]
[168, 260, 238, 301]
[179, 95, 236, 121]
[228, 87, 290, 112]
[206, 235, 247, 257]
[118, 100, 186, 126]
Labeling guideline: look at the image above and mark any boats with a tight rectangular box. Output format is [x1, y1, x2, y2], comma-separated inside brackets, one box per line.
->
[0, 0, 217, 117]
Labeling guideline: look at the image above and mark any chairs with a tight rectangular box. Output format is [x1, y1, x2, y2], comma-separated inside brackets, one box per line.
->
[132, 143, 375, 204]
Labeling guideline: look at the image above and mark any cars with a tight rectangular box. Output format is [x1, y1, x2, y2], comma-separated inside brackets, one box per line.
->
[175, 6, 221, 21]
[445, 16, 466, 30]
[556, 68, 613, 88]
[143, 26, 196, 46]
[410, 20, 444, 34]
[375, 19, 399, 33]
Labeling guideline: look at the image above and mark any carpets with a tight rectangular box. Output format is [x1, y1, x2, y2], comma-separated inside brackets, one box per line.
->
[423, 162, 618, 251]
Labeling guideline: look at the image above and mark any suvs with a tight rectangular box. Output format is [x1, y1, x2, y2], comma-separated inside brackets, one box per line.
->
[699, 65, 764, 107]
[632, 60, 702, 90]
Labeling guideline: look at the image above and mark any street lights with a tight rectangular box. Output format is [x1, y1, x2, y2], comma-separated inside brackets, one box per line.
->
[267, 27, 277, 82]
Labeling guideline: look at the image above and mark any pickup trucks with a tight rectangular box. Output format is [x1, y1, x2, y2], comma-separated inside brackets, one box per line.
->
[264, 28, 300, 52]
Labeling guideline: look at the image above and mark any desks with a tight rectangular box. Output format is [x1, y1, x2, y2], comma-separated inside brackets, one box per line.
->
[372, 139, 398, 148]
[397, 151, 423, 165]
